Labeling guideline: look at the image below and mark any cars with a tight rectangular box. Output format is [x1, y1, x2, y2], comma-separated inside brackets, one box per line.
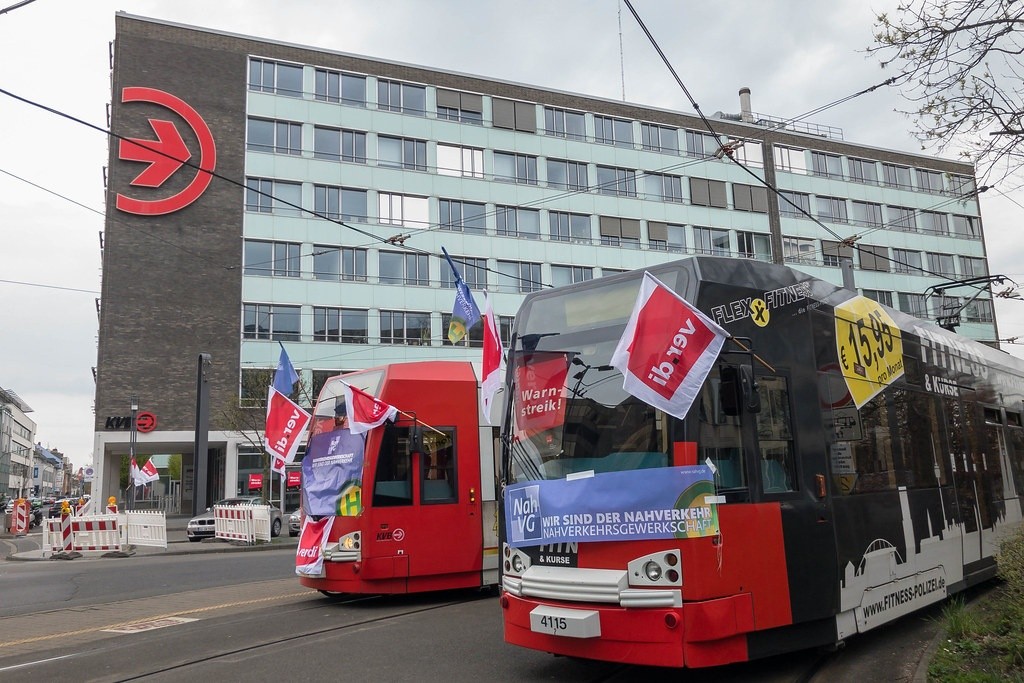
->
[6, 495, 90, 528]
[187, 496, 282, 543]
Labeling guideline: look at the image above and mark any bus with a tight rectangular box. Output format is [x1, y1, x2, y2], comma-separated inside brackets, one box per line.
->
[499, 256, 1024, 670]
[296, 360, 505, 601]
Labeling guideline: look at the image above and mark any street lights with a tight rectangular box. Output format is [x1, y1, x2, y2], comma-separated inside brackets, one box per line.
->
[40, 464, 52, 508]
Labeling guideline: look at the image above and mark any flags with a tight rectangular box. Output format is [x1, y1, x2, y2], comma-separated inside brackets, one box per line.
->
[129, 451, 147, 488]
[445, 277, 482, 345]
[271, 456, 286, 477]
[135, 459, 159, 486]
[609, 269, 731, 420]
[482, 288, 506, 425]
[272, 347, 300, 396]
[345, 383, 397, 435]
[264, 387, 312, 465]
